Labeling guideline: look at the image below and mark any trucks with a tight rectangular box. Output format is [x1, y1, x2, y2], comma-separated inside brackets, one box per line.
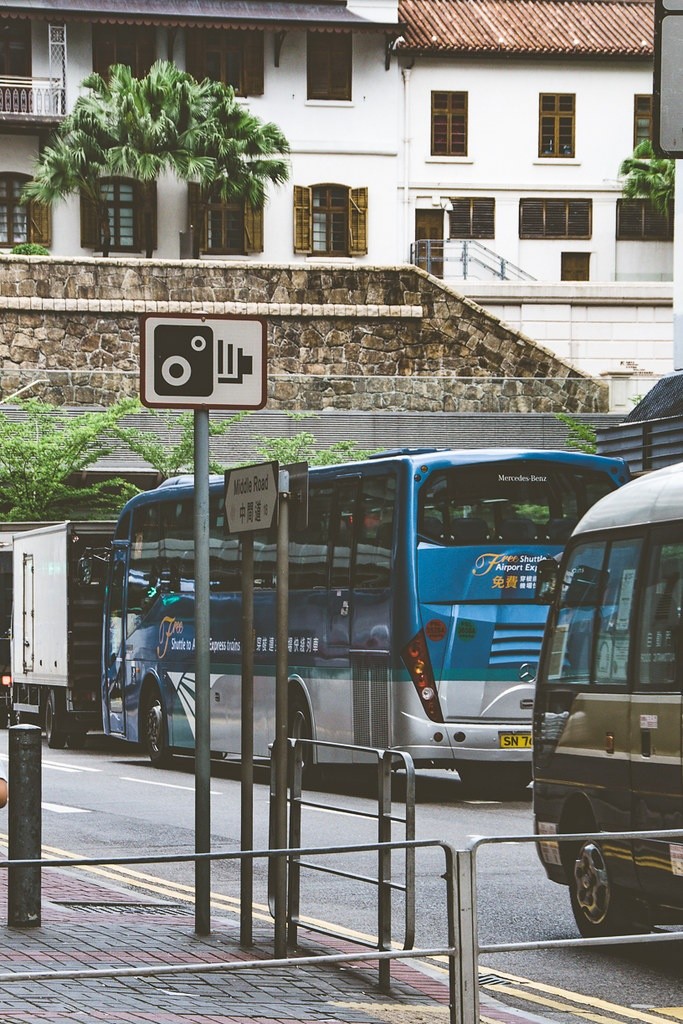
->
[1, 518, 120, 750]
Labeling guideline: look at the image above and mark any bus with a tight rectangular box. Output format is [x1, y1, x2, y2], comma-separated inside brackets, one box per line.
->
[99, 446, 640, 799]
[529, 458, 683, 947]
